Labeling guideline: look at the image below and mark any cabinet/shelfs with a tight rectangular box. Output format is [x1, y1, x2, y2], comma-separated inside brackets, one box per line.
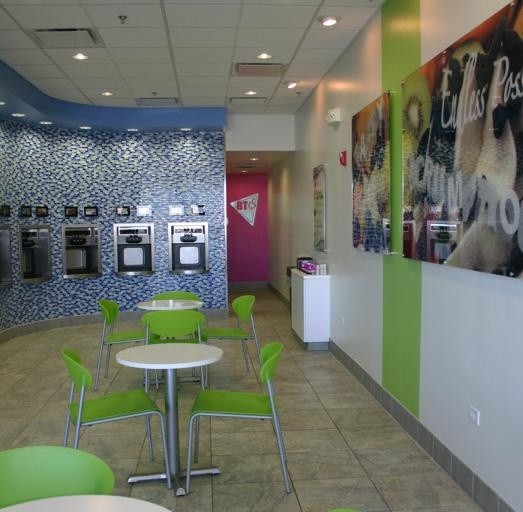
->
[290, 268, 329, 344]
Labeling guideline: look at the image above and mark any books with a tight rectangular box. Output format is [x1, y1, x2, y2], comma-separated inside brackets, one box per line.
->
[323, 263, 327, 275]
[315, 264, 319, 275]
[320, 264, 323, 275]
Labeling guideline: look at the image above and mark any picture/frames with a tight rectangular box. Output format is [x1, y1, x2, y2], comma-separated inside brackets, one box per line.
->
[0, 204, 207, 219]
